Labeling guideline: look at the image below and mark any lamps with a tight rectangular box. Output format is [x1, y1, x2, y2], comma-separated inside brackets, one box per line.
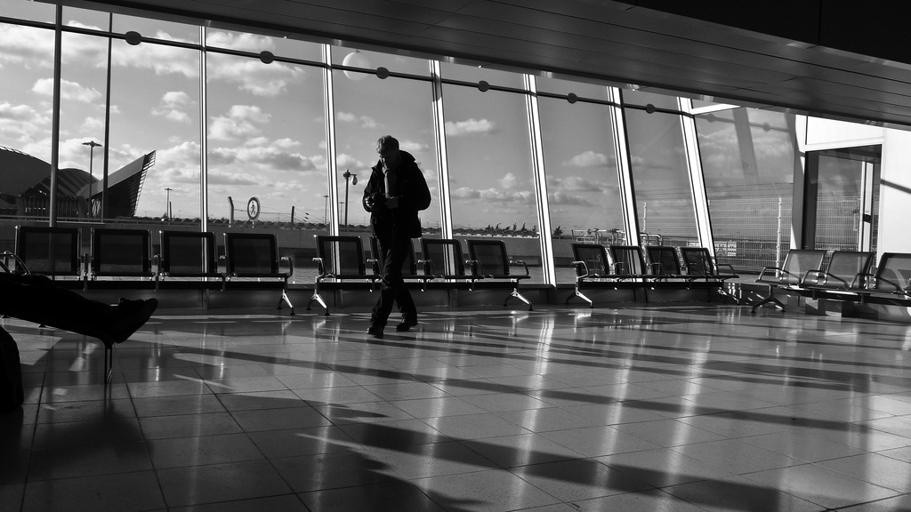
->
[342, 50, 370, 81]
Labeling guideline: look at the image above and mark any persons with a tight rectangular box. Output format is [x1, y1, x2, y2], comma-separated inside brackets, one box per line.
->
[0, 270, 160, 344]
[360, 134, 432, 338]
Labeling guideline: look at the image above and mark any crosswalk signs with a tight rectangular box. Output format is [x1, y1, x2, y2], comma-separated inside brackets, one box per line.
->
[247, 197, 260, 219]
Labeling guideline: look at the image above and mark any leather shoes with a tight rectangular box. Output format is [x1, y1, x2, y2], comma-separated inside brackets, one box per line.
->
[396, 317, 417, 332]
[365, 326, 383, 339]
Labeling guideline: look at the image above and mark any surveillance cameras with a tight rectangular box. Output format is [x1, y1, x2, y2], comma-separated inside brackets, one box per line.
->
[351, 176, 358, 186]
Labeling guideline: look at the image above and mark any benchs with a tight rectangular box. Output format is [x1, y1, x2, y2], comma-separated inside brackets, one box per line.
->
[306, 235, 533, 315]
[566, 244, 740, 308]
[751, 249, 911, 314]
[1, 226, 295, 328]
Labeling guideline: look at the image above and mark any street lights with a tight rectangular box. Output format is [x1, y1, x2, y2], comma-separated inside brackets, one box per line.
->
[163, 188, 172, 218]
[322, 170, 357, 228]
[83, 141, 102, 218]
